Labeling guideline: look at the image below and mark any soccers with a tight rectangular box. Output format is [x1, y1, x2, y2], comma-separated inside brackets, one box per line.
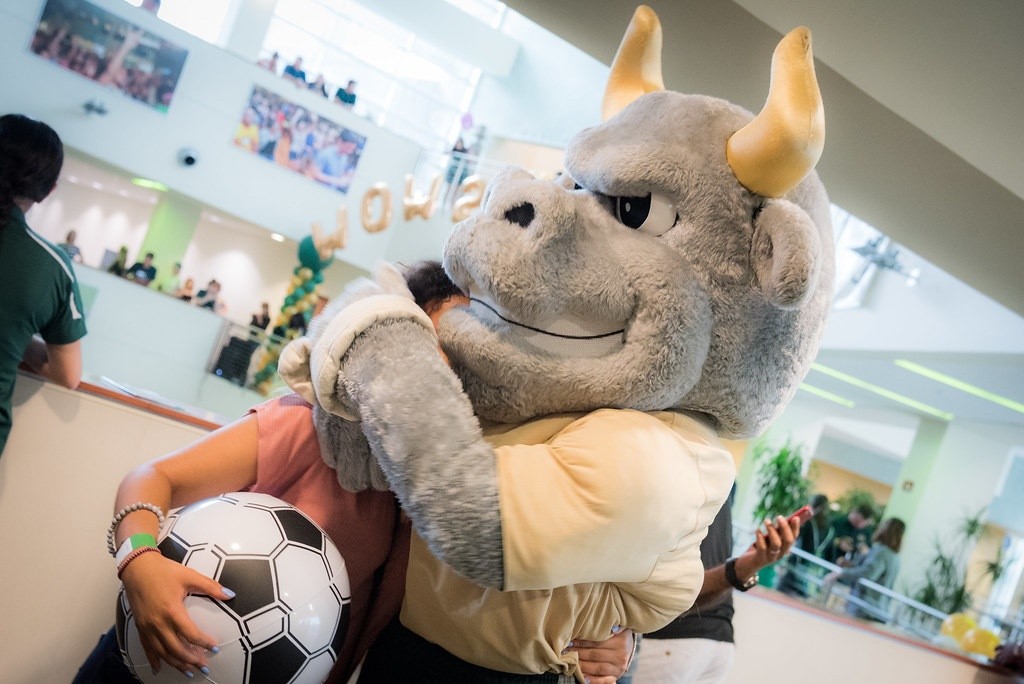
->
[114, 491, 352, 684]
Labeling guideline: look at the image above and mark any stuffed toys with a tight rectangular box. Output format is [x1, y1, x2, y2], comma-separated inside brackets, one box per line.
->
[278, 4, 833, 684]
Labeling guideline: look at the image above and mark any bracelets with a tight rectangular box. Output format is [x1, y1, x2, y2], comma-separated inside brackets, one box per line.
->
[117, 546, 161, 581]
[106, 502, 165, 557]
[115, 533, 158, 570]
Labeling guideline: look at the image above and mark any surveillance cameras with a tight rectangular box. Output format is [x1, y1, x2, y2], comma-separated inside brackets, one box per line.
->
[183, 151, 196, 165]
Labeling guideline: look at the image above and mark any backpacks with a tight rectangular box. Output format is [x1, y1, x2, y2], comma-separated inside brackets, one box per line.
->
[794, 517, 835, 595]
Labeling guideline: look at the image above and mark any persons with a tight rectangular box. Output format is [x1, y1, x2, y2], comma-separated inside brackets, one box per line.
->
[72, 262, 632, 684]
[625, 472, 800, 684]
[0, 113, 88, 457]
[777, 493, 906, 627]
[58, 229, 271, 337]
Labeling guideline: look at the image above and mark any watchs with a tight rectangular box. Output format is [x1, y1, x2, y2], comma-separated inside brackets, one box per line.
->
[724, 556, 760, 592]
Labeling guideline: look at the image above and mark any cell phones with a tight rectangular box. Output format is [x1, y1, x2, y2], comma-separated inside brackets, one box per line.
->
[753, 506, 815, 549]
